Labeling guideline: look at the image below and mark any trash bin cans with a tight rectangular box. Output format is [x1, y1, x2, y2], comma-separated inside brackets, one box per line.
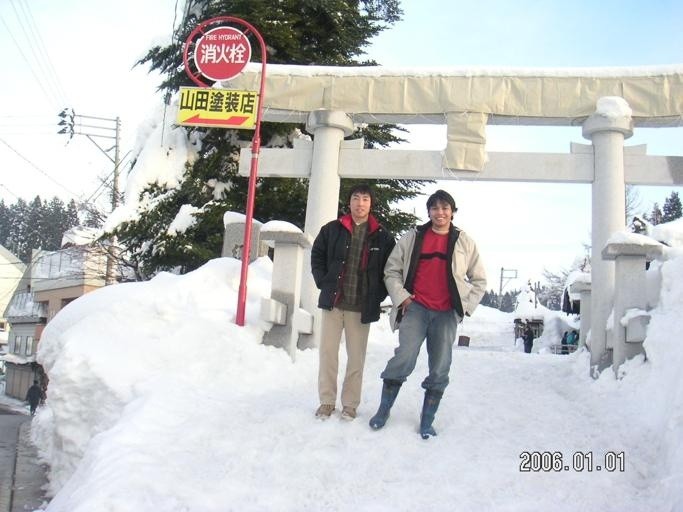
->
[458, 336, 469, 346]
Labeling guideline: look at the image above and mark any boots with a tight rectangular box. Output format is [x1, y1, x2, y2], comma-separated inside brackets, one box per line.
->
[420, 389, 442, 439]
[370, 378, 403, 429]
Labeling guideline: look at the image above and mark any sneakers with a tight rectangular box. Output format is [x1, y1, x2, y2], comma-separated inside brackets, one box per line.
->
[316, 405, 336, 419]
[341, 406, 357, 421]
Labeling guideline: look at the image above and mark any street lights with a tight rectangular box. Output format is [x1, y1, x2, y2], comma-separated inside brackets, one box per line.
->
[57, 107, 122, 286]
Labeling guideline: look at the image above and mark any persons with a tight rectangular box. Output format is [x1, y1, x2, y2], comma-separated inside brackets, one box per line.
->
[25, 380, 44, 416]
[369, 190, 487, 440]
[569, 330, 578, 352]
[521, 326, 533, 353]
[311, 184, 396, 424]
[561, 331, 569, 355]
